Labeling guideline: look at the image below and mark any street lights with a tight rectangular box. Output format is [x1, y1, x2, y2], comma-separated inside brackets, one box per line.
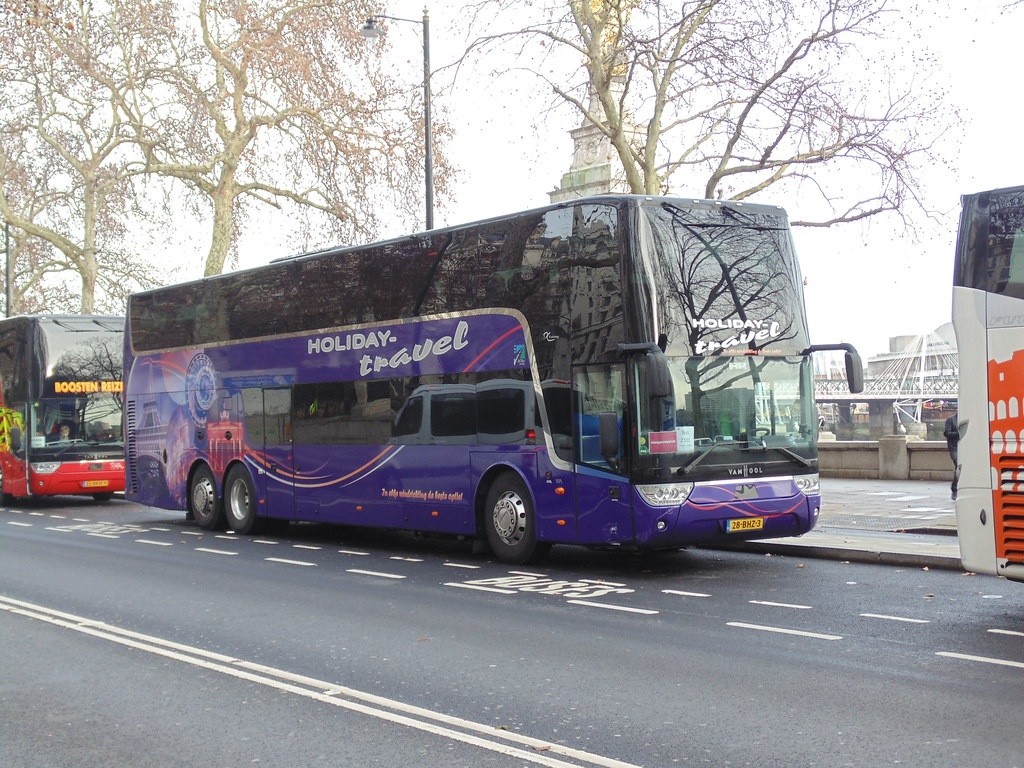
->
[1, 221, 11, 320]
[361, 5, 434, 232]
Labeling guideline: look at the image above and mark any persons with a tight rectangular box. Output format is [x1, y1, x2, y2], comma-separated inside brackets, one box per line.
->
[943, 414, 958, 501]
[48, 425, 71, 442]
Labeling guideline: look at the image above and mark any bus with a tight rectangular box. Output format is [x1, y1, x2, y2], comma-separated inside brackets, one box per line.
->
[952, 185, 1024, 585]
[0, 316, 129, 505]
[122, 191, 865, 567]
